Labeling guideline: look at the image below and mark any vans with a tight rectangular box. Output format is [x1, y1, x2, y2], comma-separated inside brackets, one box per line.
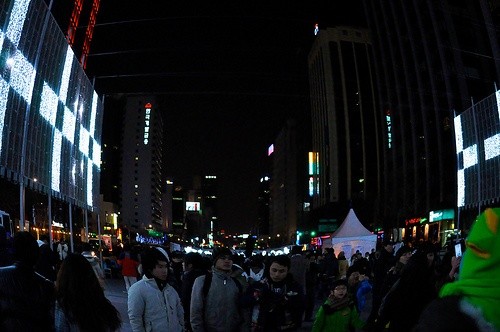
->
[88, 238, 112, 263]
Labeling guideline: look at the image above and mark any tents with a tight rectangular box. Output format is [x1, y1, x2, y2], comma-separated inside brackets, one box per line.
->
[321, 208, 378, 267]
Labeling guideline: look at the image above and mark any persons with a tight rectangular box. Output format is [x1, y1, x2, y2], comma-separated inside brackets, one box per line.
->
[0, 226, 122, 332]
[117, 239, 319, 332]
[311, 239, 481, 332]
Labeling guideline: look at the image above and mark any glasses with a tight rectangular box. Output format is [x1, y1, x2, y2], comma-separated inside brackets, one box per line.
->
[334, 286, 346, 291]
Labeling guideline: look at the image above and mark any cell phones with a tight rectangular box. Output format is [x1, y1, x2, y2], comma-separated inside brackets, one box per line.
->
[454, 243, 463, 259]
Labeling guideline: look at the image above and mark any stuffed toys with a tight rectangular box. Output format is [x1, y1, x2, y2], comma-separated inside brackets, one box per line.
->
[437, 208, 500, 332]
